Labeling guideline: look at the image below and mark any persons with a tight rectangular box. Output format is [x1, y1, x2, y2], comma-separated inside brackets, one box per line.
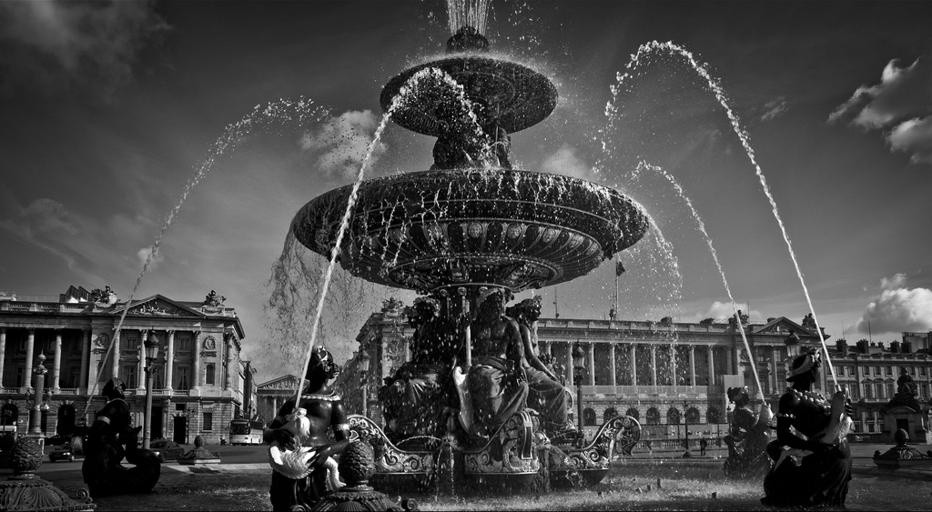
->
[762, 349, 855, 510]
[176, 435, 219, 465]
[723, 385, 770, 456]
[78, 377, 155, 494]
[260, 345, 352, 511]
[380, 283, 574, 444]
[699, 437, 707, 456]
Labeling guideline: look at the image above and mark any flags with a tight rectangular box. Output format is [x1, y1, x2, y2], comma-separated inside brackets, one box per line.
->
[616, 255, 626, 277]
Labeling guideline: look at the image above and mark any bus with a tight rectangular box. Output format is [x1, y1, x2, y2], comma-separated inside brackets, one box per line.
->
[226, 418, 265, 447]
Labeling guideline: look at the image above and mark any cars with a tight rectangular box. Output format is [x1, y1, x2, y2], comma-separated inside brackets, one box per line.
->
[48, 444, 84, 463]
[149, 439, 186, 462]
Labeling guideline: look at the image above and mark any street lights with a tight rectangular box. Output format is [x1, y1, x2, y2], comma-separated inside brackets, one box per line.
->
[679, 393, 697, 458]
[725, 405, 733, 455]
[143, 325, 164, 451]
[710, 406, 725, 447]
[571, 338, 587, 437]
